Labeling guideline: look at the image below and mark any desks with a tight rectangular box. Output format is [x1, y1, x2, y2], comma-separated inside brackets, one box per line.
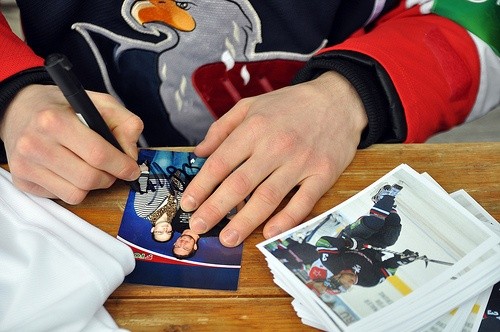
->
[54, 142, 500, 332]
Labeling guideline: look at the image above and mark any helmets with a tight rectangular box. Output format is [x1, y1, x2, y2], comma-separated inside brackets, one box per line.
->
[322, 276, 348, 296]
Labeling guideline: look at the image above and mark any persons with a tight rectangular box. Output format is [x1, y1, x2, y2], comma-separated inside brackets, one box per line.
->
[0, 0, 500, 248]
[270, 184, 419, 310]
[134, 151, 247, 259]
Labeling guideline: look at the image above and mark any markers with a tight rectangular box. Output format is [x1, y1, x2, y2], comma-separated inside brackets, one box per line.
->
[43, 53, 144, 196]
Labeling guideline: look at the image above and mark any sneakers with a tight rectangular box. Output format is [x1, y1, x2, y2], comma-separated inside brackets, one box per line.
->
[371, 180, 404, 204]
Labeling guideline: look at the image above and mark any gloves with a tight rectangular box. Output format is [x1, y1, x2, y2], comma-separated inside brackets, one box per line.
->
[347, 236, 370, 252]
[394, 249, 419, 266]
